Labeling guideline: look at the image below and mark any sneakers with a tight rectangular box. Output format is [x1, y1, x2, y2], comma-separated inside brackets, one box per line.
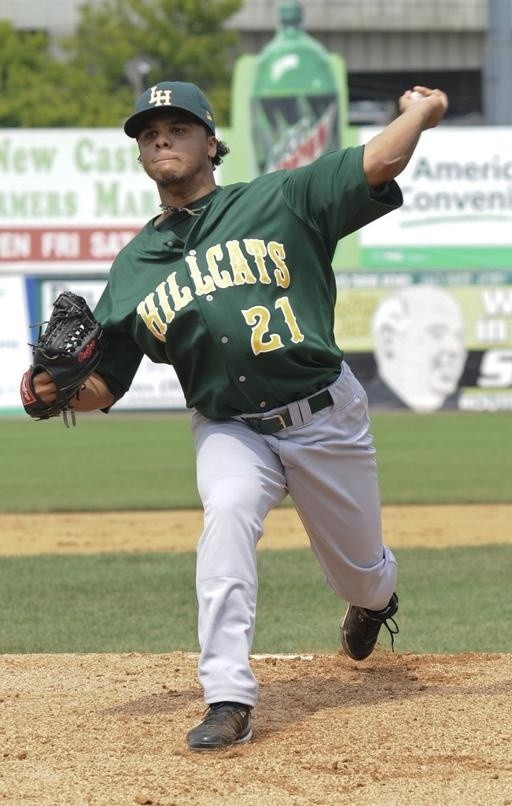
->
[187, 701, 253, 751]
[340, 592, 399, 660]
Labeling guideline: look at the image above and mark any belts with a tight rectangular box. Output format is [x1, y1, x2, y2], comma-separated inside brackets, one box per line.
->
[241, 389, 334, 435]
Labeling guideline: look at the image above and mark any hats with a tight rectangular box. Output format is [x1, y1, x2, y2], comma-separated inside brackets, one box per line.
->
[123, 80, 216, 138]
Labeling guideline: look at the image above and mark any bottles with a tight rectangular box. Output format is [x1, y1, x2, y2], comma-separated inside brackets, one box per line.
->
[247, 0, 343, 176]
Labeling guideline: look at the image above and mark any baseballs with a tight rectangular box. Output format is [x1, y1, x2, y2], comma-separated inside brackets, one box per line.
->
[409, 90, 425, 102]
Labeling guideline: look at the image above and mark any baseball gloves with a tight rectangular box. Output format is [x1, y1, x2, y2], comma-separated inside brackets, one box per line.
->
[20, 290, 111, 420]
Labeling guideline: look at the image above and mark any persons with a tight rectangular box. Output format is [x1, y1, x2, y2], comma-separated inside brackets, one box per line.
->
[19, 81, 449, 751]
